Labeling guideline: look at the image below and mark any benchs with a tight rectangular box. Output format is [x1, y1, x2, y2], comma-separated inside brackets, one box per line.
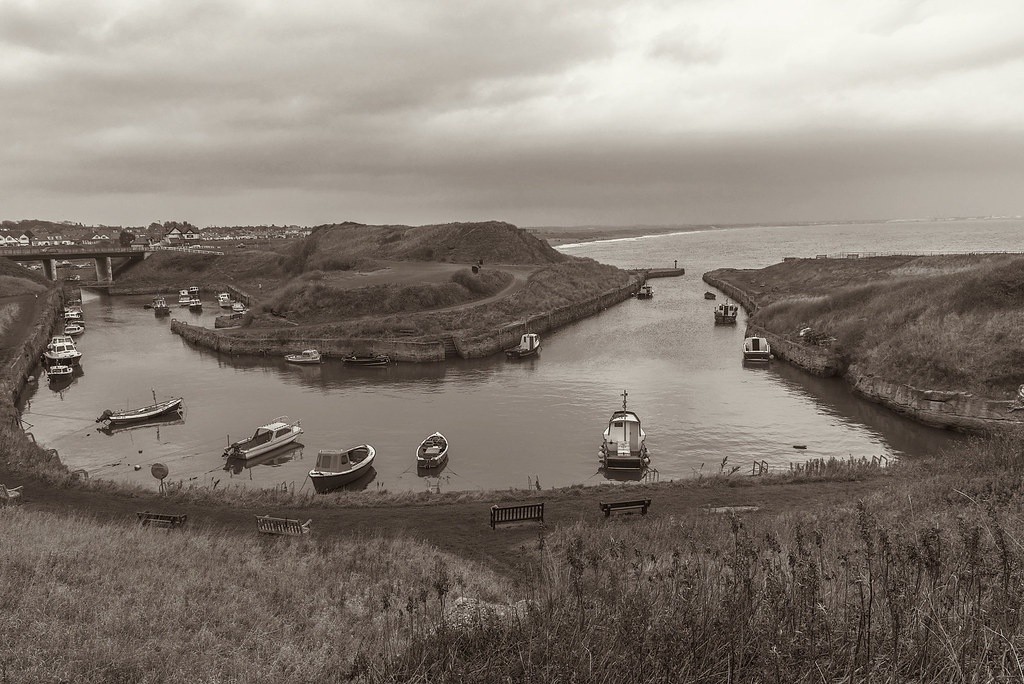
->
[137, 509, 187, 529]
[600, 497, 651, 518]
[256, 515, 313, 538]
[0, 484, 24, 507]
[490, 502, 544, 531]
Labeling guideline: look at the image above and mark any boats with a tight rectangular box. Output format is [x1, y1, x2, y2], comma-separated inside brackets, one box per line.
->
[712, 298, 737, 324]
[601, 388, 647, 472]
[341, 352, 389, 367]
[741, 334, 770, 363]
[307, 443, 376, 492]
[41, 285, 246, 378]
[108, 389, 184, 425]
[640, 279, 654, 299]
[283, 350, 323, 366]
[415, 431, 448, 469]
[504, 333, 542, 358]
[224, 416, 303, 459]
[703, 290, 716, 301]
